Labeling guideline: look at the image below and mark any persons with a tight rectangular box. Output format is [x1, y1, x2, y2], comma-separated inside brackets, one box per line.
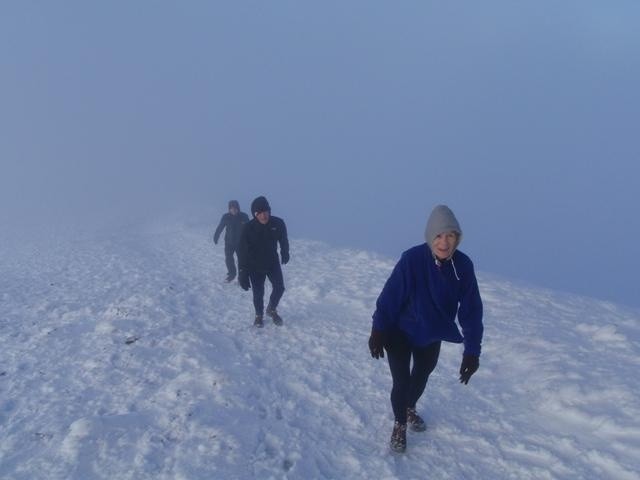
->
[366, 204, 486, 454]
[213, 200, 253, 284]
[237, 195, 291, 328]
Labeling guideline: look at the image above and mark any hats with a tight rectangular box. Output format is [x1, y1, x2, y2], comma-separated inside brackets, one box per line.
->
[228, 200, 241, 212]
[251, 196, 271, 218]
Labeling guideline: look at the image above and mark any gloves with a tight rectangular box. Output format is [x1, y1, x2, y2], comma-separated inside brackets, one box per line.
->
[368, 329, 386, 359]
[460, 355, 480, 384]
[237, 275, 251, 291]
[282, 253, 290, 264]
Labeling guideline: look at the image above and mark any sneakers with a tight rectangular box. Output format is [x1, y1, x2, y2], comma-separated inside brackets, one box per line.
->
[266, 311, 283, 326]
[254, 316, 263, 328]
[391, 419, 406, 454]
[225, 276, 233, 282]
[406, 406, 426, 431]
